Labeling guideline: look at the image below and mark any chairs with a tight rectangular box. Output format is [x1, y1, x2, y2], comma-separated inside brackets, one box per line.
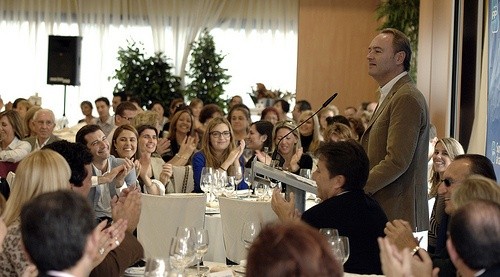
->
[135, 192, 207, 265]
[218, 195, 278, 264]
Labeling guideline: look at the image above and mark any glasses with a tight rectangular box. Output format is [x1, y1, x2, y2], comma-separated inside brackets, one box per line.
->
[440, 179, 456, 187]
[208, 131, 231, 138]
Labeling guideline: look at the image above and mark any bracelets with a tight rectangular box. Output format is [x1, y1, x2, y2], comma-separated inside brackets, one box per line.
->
[146, 182, 153, 188]
[410, 246, 419, 256]
[175, 154, 187, 161]
[219, 166, 227, 171]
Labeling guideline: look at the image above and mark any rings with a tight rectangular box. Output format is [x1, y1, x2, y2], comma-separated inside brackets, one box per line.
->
[115, 240, 120, 246]
[98, 247, 105, 255]
[114, 236, 118, 240]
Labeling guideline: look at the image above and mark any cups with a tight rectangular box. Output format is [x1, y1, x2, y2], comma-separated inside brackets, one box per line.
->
[144, 257, 169, 277]
[329, 237, 349, 265]
[241, 222, 262, 247]
[300, 168, 311, 179]
[242, 148, 253, 162]
[320, 228, 339, 242]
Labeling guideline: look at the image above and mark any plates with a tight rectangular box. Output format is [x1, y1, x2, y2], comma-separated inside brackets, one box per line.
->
[123, 267, 145, 277]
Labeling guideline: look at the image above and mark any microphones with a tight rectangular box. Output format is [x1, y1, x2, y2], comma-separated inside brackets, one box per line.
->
[270, 93, 338, 166]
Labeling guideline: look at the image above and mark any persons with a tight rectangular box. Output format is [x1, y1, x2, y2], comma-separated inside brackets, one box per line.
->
[245, 137, 500, 277]
[356, 28, 431, 233]
[19, 190, 98, 277]
[0, 95, 378, 276]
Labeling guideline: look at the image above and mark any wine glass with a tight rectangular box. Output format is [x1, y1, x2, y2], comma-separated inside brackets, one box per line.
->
[168, 227, 209, 277]
[200, 166, 268, 206]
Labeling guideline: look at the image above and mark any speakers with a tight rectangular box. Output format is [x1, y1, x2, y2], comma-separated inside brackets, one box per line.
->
[47, 35, 83, 85]
